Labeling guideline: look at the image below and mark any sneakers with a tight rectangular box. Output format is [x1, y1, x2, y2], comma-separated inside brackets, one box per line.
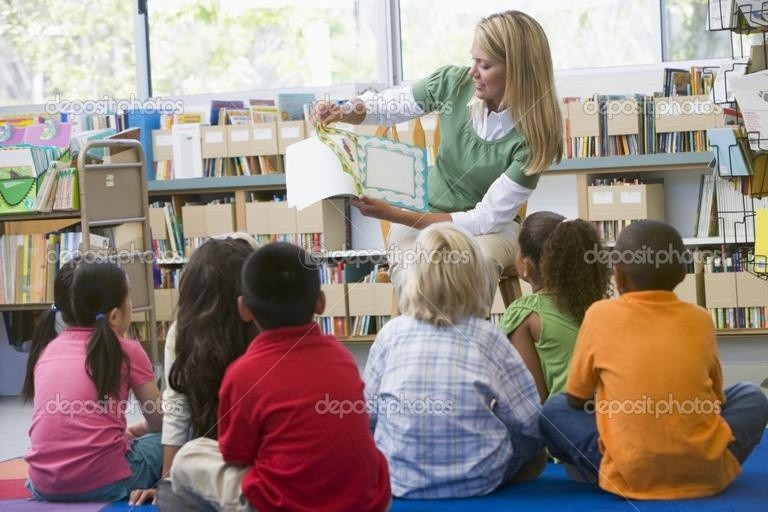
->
[508, 448, 547, 483]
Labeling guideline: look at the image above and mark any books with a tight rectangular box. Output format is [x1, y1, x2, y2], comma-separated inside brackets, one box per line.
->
[1, 66, 767, 353]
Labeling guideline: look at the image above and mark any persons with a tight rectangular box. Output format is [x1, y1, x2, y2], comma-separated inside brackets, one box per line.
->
[128, 232, 261, 507]
[18, 256, 162, 503]
[361, 221, 547, 500]
[170, 243, 392, 512]
[499, 211, 616, 466]
[312, 11, 567, 323]
[539, 220, 767, 502]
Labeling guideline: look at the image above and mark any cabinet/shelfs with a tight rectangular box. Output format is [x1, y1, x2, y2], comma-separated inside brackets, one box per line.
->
[148, 153, 768, 345]
[0, 137, 159, 393]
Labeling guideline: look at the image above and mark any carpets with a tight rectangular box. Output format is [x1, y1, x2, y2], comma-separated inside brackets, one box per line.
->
[1, 419, 766, 512]
